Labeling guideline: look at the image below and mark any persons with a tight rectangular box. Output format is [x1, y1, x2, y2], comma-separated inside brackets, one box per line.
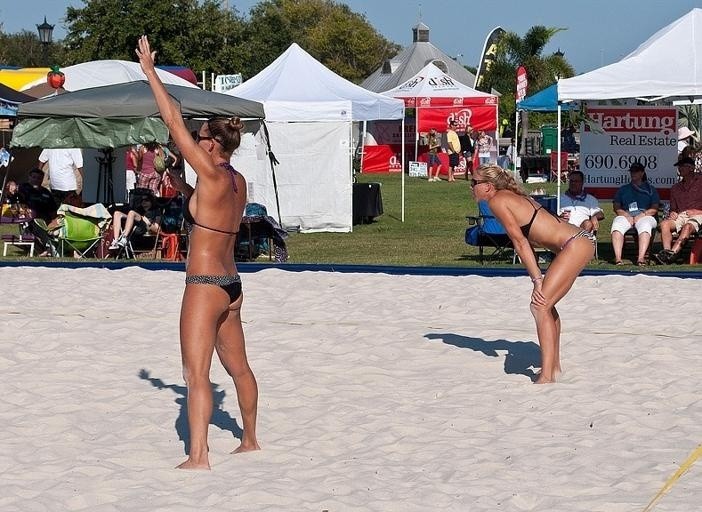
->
[560, 171, 605, 233]
[505, 137, 516, 164]
[652, 125, 702, 265]
[447, 121, 461, 181]
[473, 128, 493, 165]
[470, 165, 597, 384]
[134, 35, 261, 471]
[7, 147, 182, 259]
[610, 162, 660, 267]
[426, 128, 442, 183]
[462, 125, 474, 180]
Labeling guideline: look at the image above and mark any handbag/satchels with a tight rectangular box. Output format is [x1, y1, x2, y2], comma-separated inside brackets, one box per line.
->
[152, 153, 167, 173]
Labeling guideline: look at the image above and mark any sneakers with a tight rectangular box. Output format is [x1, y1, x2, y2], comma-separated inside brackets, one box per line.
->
[433, 176, 441, 182]
[652, 249, 685, 265]
[426, 178, 436, 182]
[447, 175, 455, 182]
[109, 239, 120, 250]
[118, 235, 127, 248]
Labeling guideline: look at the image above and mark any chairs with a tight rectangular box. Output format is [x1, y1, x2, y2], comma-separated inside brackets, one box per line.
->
[466, 150, 701, 265]
[0, 180, 273, 265]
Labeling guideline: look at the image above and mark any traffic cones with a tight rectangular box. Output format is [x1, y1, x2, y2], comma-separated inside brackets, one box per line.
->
[164, 237, 182, 261]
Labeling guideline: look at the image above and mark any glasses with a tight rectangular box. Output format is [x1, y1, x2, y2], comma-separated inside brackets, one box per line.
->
[453, 125, 457, 126]
[194, 133, 213, 143]
[470, 178, 489, 188]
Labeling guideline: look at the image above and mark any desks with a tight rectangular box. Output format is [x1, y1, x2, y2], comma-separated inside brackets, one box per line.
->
[352, 181, 385, 226]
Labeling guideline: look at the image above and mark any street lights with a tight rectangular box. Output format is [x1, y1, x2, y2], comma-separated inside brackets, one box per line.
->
[31, 11, 57, 66]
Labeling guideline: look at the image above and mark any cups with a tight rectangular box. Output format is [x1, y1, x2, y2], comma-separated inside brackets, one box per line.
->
[564, 209, 571, 220]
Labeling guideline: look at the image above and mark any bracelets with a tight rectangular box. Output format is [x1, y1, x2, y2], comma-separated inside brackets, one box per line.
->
[532, 277, 541, 283]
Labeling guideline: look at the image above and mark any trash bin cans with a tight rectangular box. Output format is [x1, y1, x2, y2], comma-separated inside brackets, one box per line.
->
[541, 127, 557, 155]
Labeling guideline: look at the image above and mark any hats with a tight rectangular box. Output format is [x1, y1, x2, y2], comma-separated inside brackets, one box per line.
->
[677, 127, 695, 141]
[673, 158, 695, 166]
[628, 163, 643, 171]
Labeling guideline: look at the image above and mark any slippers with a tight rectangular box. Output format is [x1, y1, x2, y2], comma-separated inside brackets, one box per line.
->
[615, 261, 624, 266]
[637, 261, 646, 268]
